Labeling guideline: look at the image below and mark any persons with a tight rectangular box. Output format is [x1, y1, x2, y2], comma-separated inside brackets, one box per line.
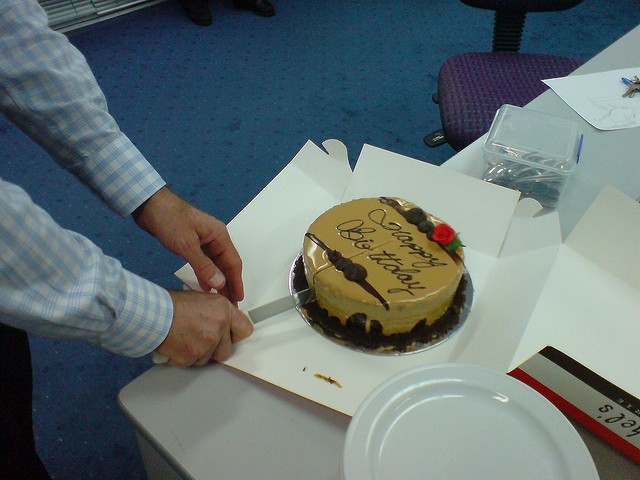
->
[180, 0, 275, 26]
[0, 0, 255, 480]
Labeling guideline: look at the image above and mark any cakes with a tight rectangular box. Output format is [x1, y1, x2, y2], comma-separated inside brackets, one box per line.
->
[292, 196, 469, 356]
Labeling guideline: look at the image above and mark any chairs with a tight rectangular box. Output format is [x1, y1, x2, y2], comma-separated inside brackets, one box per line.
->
[423, 0, 586, 151]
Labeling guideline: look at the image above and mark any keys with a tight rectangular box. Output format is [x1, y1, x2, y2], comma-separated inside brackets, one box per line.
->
[622, 76, 640, 98]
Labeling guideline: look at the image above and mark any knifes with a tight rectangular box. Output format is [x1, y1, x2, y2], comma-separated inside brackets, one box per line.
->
[150, 288, 316, 365]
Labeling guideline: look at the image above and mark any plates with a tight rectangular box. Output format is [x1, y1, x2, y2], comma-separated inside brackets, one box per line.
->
[340, 364, 601, 480]
